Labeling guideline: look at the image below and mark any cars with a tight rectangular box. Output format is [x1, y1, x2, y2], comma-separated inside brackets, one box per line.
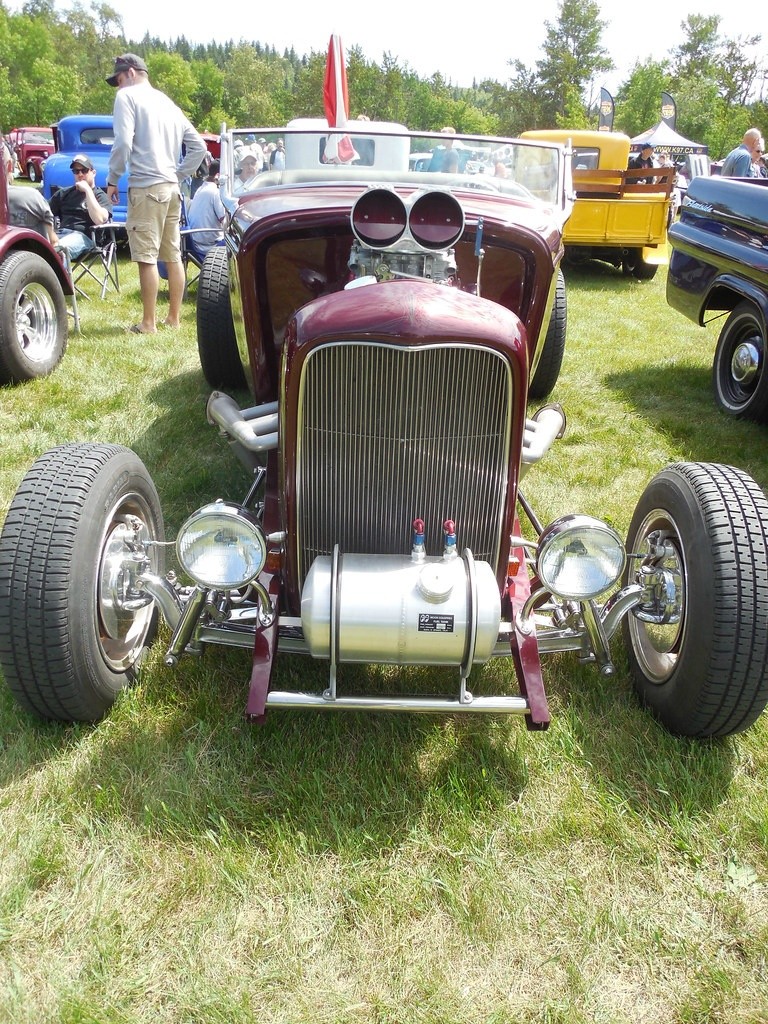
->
[9, 127, 59, 181]
[39, 115, 183, 231]
[0, 140, 75, 384]
[1, 117, 768, 763]
[410, 152, 435, 172]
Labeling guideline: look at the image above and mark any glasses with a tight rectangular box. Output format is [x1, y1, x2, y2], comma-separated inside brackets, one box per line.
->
[756, 150, 763, 153]
[72, 168, 89, 174]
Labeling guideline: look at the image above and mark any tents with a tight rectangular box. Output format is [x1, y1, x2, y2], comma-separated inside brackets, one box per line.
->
[629, 121, 707, 155]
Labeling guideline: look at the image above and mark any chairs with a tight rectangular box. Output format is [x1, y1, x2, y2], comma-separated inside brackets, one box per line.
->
[48, 187, 120, 301]
[177, 191, 223, 300]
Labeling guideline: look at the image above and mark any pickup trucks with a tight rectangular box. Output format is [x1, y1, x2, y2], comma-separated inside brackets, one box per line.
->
[661, 178, 768, 419]
[513, 128, 673, 277]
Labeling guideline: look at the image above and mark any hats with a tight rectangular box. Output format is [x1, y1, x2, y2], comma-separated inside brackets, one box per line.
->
[209, 158, 220, 176]
[642, 142, 657, 147]
[234, 167, 242, 175]
[70, 154, 93, 170]
[239, 150, 258, 162]
[258, 138, 266, 143]
[105, 53, 148, 86]
[234, 139, 244, 146]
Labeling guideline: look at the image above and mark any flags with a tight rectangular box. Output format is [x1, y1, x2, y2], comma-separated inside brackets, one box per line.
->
[323, 34, 360, 165]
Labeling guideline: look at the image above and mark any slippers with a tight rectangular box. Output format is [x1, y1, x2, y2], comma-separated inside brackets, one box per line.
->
[130, 325, 143, 334]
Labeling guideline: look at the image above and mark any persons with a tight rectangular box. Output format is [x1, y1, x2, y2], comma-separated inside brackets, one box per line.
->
[627, 143, 656, 184]
[4, 145, 58, 245]
[47, 153, 113, 258]
[440, 127, 461, 173]
[188, 161, 228, 256]
[655, 151, 681, 223]
[235, 154, 258, 189]
[233, 134, 285, 174]
[104, 53, 207, 334]
[720, 128, 768, 178]
